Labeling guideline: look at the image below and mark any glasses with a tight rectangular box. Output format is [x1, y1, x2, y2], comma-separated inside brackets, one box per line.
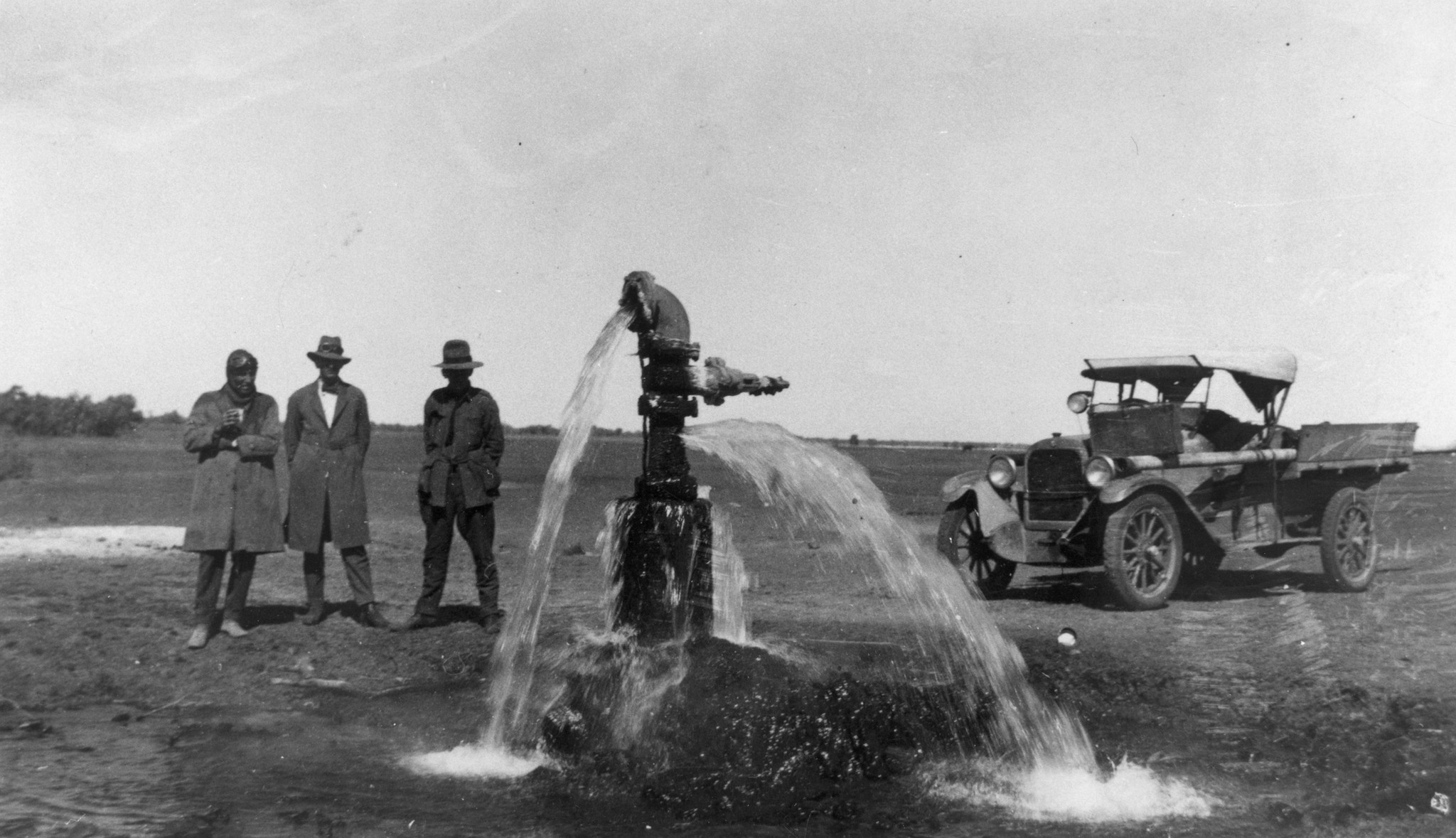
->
[228, 355, 257, 364]
[319, 344, 344, 355]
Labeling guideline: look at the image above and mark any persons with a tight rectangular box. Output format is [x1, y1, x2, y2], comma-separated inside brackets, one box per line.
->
[282, 335, 386, 627]
[389, 340, 505, 633]
[179, 350, 287, 647]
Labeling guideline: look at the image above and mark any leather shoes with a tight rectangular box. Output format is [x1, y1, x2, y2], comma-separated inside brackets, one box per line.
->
[302, 603, 325, 625]
[408, 613, 440, 627]
[483, 614, 501, 633]
[357, 603, 389, 627]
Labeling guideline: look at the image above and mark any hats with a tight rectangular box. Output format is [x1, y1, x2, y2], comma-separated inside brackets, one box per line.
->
[307, 335, 352, 365]
[434, 339, 484, 369]
[227, 349, 258, 368]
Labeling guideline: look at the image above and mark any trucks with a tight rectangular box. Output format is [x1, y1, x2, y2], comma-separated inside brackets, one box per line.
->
[936, 346, 1427, 612]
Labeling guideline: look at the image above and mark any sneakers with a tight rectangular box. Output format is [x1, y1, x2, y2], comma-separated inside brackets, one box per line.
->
[220, 617, 248, 638]
[187, 624, 215, 647]
